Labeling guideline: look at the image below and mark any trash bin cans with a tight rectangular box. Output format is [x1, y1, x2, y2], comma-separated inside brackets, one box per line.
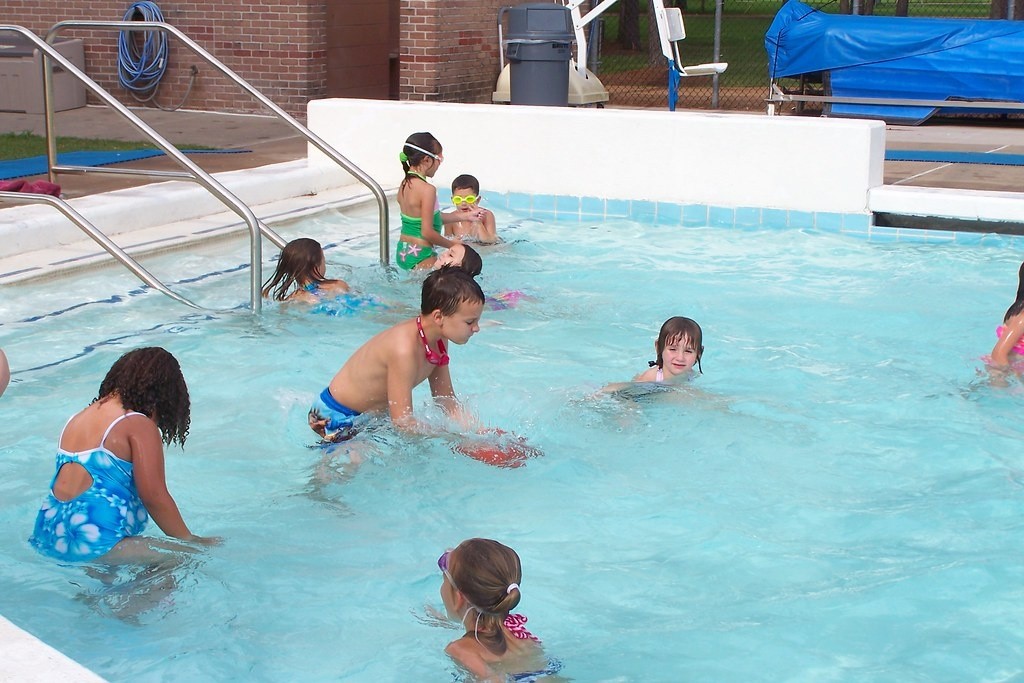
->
[505, 4, 576, 105]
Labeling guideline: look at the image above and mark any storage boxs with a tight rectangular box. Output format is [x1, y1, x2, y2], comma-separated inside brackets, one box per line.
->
[0, 35, 86, 114]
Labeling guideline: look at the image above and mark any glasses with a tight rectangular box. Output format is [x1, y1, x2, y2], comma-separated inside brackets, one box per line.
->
[438, 552, 450, 571]
[439, 156, 444, 162]
[425, 351, 450, 367]
[452, 194, 479, 204]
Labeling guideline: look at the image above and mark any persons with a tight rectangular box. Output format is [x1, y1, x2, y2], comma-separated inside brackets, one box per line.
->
[35, 347, 193, 551]
[991, 262, 1024, 362]
[310, 265, 487, 422]
[439, 538, 541, 673]
[396, 132, 486, 270]
[444, 175, 495, 238]
[433, 243, 482, 275]
[261, 238, 348, 302]
[642, 316, 704, 382]
[0, 349, 10, 396]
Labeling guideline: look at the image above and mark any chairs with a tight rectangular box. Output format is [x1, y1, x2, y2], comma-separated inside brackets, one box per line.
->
[663, 7, 728, 77]
